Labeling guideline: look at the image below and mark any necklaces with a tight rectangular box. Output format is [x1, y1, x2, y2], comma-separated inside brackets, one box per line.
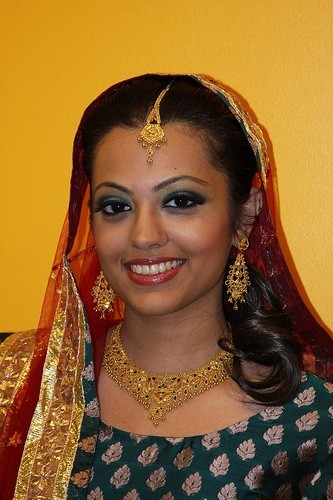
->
[101, 322, 244, 426]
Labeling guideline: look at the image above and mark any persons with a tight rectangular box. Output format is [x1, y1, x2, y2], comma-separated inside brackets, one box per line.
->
[0, 73, 333, 500]
